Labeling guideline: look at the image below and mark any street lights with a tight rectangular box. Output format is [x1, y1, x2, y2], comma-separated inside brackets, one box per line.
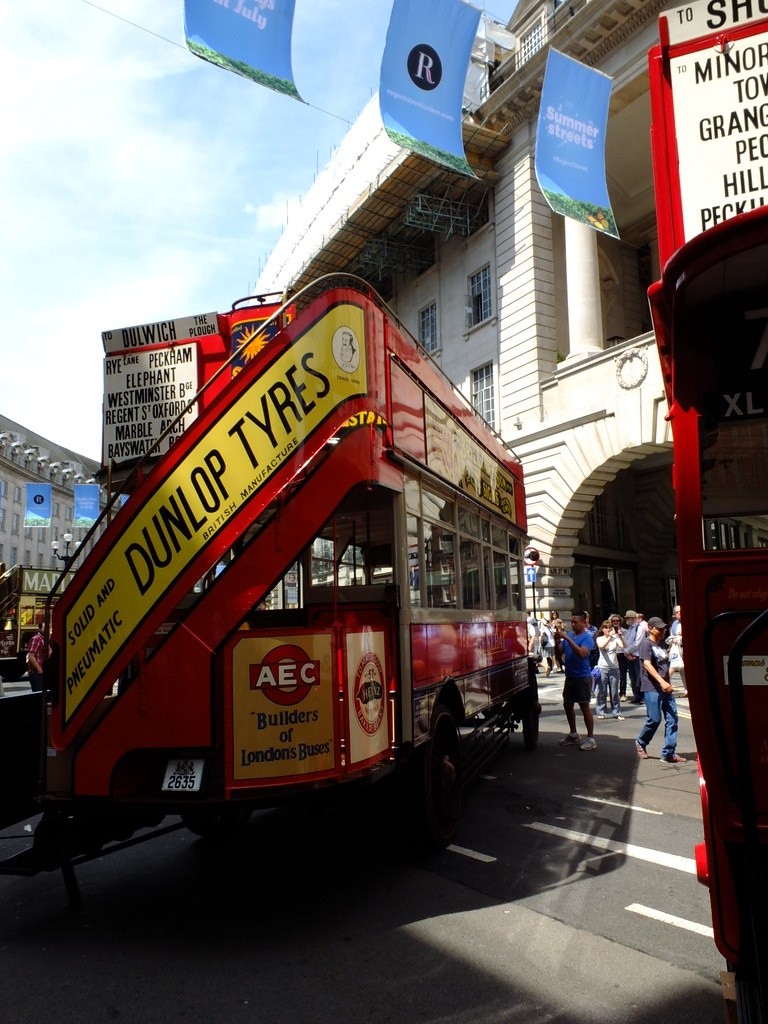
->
[50, 526, 83, 572]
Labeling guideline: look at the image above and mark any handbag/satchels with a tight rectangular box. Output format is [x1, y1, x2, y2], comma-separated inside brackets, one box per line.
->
[541, 633, 549, 648]
[665, 636, 684, 671]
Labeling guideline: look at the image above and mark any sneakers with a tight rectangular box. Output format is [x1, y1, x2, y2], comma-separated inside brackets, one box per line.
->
[559, 734, 582, 745]
[580, 737, 596, 750]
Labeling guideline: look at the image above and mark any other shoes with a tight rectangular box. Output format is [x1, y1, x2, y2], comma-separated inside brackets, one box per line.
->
[633, 740, 648, 759]
[661, 755, 687, 763]
[545, 668, 553, 676]
[619, 695, 626, 701]
[616, 716, 626, 721]
[597, 715, 605, 719]
[607, 696, 611, 702]
[679, 694, 688, 698]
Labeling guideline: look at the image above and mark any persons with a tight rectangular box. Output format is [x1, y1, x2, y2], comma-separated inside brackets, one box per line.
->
[606, 613, 628, 702]
[28, 622, 50, 692]
[635, 617, 686, 764]
[639, 614, 649, 637]
[539, 611, 565, 677]
[554, 609, 597, 750]
[624, 610, 646, 704]
[584, 611, 598, 698]
[527, 611, 538, 655]
[670, 605, 688, 699]
[593, 621, 626, 721]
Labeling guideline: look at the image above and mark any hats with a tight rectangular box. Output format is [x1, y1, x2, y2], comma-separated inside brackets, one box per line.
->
[624, 610, 638, 618]
[648, 616, 667, 629]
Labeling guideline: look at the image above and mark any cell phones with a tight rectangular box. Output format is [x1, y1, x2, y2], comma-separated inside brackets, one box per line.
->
[614, 625, 618, 634]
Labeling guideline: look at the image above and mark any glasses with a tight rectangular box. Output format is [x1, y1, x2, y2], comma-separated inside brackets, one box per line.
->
[612, 619, 620, 622]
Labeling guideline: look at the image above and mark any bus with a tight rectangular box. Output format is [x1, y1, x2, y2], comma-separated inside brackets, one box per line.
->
[642, 202, 767, 1024]
[36, 268, 540, 853]
[0, 561, 73, 681]
